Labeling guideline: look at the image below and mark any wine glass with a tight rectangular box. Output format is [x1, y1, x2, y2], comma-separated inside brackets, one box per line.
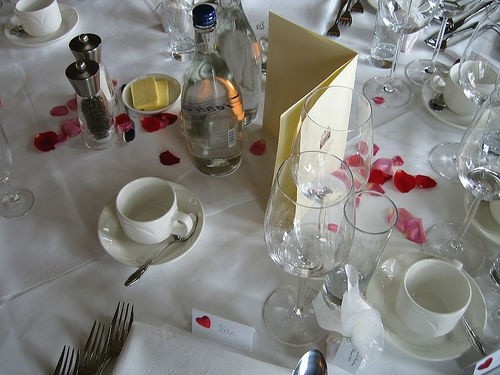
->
[423, 88, 500, 272]
[291, 84, 376, 280]
[0, 123, 35, 218]
[363, 0, 500, 269]
[260, 150, 355, 347]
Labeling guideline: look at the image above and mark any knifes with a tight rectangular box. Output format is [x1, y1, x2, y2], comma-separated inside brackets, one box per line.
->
[426, 0, 495, 41]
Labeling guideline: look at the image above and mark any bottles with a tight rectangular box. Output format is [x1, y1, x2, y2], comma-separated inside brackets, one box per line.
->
[213, 0, 264, 128]
[179, 4, 244, 177]
[69, 33, 118, 133]
[64, 59, 120, 150]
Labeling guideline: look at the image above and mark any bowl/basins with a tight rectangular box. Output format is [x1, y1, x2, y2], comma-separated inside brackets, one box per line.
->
[121, 73, 182, 116]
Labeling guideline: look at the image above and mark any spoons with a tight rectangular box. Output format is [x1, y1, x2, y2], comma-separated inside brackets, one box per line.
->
[125, 212, 198, 287]
[291, 349, 328, 375]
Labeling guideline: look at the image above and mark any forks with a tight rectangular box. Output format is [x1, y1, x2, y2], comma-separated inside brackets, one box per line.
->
[52, 299, 135, 375]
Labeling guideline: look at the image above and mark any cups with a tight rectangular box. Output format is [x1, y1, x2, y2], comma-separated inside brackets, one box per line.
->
[442, 59, 499, 116]
[161, 0, 196, 54]
[481, 256, 500, 352]
[14, 0, 62, 37]
[320, 190, 398, 313]
[116, 176, 193, 244]
[397, 257, 473, 338]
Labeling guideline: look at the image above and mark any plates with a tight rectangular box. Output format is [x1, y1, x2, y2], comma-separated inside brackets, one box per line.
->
[4, 3, 80, 47]
[422, 75, 475, 129]
[97, 179, 205, 267]
[365, 251, 488, 361]
[463, 189, 500, 244]
